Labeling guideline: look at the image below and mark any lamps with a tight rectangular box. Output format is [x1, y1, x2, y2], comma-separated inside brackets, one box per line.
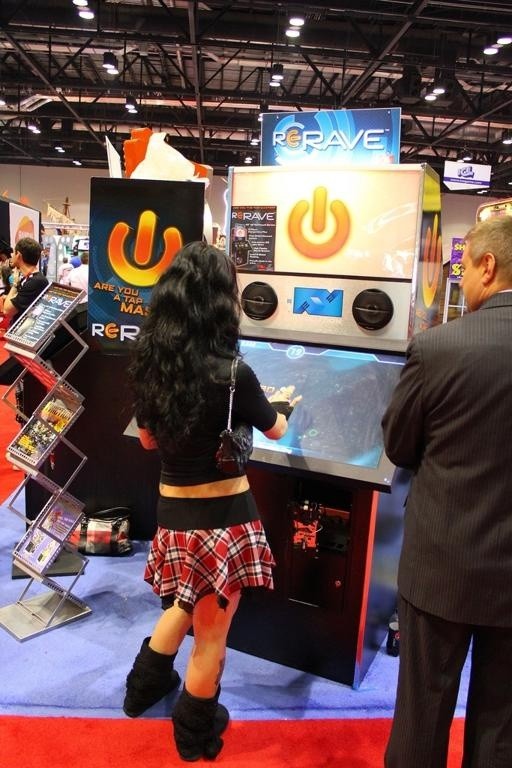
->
[28, 120, 42, 134]
[54, 142, 66, 153]
[72, 157, 82, 166]
[125, 95, 138, 114]
[269, 64, 284, 87]
[71, 0, 96, 20]
[285, 13, 306, 38]
[483, 29, 512, 56]
[501, 129, 512, 145]
[424, 81, 446, 101]
[102, 52, 119, 75]
[244, 112, 266, 165]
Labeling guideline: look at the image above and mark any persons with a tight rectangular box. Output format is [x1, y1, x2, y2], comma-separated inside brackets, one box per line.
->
[119, 235, 307, 765]
[375, 206, 510, 766]
[0, 237, 89, 329]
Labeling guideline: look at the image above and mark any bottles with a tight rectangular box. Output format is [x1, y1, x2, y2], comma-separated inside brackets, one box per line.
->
[386, 609, 400, 657]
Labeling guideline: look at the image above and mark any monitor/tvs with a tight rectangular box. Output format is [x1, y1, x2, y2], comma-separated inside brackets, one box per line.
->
[123, 326, 409, 492]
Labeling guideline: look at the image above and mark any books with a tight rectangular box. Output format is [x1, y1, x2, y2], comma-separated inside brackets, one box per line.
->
[13, 397, 79, 465]
[12, 285, 83, 348]
[17, 500, 80, 575]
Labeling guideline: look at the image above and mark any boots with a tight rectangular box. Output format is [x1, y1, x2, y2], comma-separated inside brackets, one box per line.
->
[122, 638, 182, 719]
[170, 682, 231, 762]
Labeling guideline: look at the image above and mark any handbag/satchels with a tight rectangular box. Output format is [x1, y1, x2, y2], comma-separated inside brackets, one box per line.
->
[217, 354, 255, 478]
